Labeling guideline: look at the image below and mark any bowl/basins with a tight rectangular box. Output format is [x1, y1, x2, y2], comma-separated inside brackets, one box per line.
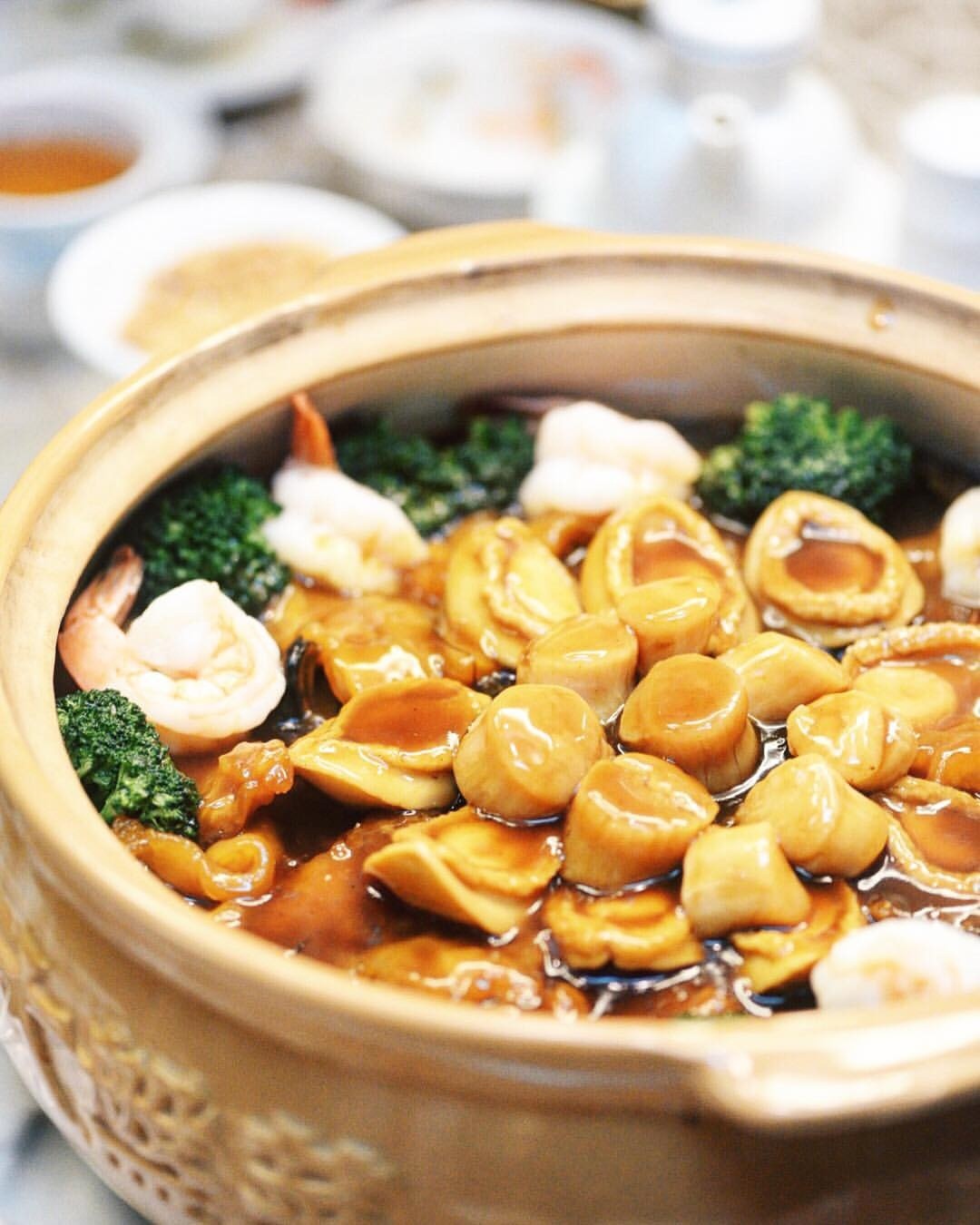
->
[1, 220, 980, 1225]
[1, 0, 980, 394]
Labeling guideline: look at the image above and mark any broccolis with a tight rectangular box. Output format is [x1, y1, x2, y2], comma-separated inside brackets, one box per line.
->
[105, 383, 917, 619]
[54, 690, 200, 835]
